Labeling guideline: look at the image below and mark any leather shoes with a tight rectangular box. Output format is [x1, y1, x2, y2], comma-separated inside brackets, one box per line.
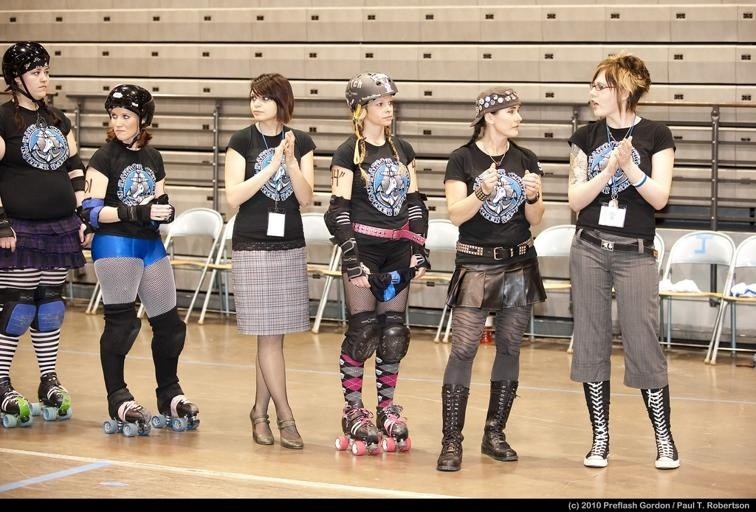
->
[277, 416, 305, 450]
[250, 406, 274, 444]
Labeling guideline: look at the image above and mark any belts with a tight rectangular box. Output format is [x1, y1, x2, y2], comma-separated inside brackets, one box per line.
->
[575, 227, 658, 258]
[456, 238, 534, 262]
[349, 220, 426, 246]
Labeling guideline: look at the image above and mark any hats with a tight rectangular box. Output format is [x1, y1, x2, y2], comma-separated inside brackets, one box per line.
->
[469, 86, 521, 127]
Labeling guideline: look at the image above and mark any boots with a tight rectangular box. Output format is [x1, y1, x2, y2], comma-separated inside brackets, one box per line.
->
[583, 381, 609, 469]
[640, 385, 680, 470]
[482, 380, 518, 462]
[437, 386, 469, 472]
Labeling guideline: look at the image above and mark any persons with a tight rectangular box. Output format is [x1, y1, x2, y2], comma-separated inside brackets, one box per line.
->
[565, 53, 681, 470]
[437, 86, 546, 472]
[223, 72, 318, 450]
[0, 40, 93, 414]
[324, 71, 431, 445]
[84, 81, 200, 423]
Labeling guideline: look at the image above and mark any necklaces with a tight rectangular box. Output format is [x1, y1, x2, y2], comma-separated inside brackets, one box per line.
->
[481, 140, 509, 167]
[33, 108, 47, 128]
[606, 122, 631, 143]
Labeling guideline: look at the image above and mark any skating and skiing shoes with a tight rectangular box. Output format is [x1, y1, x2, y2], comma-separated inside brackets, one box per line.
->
[31, 374, 73, 421]
[377, 406, 411, 453]
[153, 386, 199, 431]
[103, 389, 152, 437]
[0, 377, 34, 429]
[336, 409, 384, 455]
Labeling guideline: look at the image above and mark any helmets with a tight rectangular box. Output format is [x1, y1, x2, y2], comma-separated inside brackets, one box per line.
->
[344, 71, 397, 109]
[105, 83, 155, 125]
[0, 40, 50, 85]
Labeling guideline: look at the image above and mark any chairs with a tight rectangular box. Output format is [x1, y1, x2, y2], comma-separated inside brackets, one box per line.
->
[63, 207, 756, 367]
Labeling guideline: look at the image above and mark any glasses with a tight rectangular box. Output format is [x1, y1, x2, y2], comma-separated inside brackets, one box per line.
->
[590, 80, 616, 91]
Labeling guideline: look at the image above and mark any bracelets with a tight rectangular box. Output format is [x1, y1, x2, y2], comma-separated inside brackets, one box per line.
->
[475, 186, 492, 202]
[524, 190, 541, 204]
[632, 173, 648, 190]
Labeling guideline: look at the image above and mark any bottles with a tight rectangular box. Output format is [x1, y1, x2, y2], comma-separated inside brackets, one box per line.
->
[479, 317, 494, 345]
